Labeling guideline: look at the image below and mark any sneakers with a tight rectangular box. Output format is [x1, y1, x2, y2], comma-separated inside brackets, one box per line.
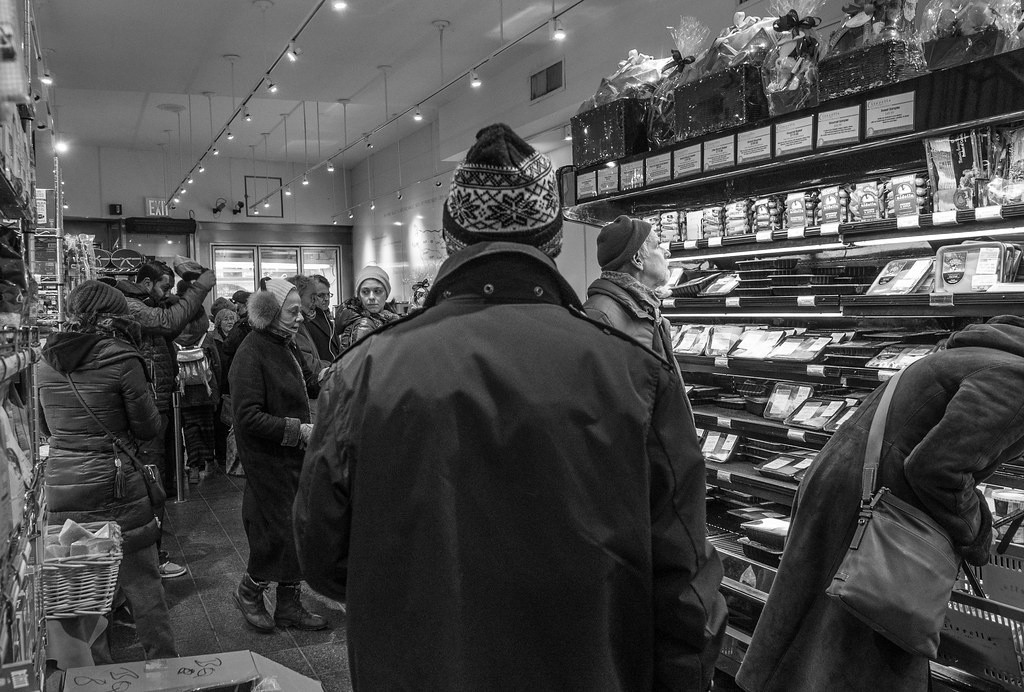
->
[164, 549, 169, 558]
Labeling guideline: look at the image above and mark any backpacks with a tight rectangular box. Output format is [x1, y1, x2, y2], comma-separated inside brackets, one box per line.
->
[172, 333, 213, 397]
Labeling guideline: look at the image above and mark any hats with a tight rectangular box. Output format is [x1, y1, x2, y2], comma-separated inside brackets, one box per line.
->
[596, 215, 652, 271]
[211, 297, 236, 318]
[258, 278, 297, 321]
[356, 266, 391, 299]
[173, 254, 213, 292]
[442, 123, 575, 260]
[232, 290, 252, 304]
[65, 280, 131, 320]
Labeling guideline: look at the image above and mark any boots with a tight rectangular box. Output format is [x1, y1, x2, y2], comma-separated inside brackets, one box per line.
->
[231, 568, 277, 633]
[273, 583, 327, 630]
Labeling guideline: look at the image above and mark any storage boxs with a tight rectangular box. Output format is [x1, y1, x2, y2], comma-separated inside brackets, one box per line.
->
[565, 9, 1009, 164]
[58, 650, 327, 692]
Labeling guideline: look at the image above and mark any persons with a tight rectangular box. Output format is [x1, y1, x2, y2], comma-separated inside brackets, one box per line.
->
[292, 122, 727, 692]
[734, 315, 1024, 692]
[98, 261, 217, 577]
[35, 281, 178, 666]
[228, 276, 329, 632]
[581, 215, 682, 375]
[173, 266, 429, 485]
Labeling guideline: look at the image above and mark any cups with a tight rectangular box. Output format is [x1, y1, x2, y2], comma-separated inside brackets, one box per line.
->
[332, 305, 338, 319]
[402, 306, 409, 316]
[395, 301, 409, 314]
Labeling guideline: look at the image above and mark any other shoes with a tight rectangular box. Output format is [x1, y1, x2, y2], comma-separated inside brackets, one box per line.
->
[202, 459, 218, 479]
[158, 561, 187, 577]
[188, 467, 199, 483]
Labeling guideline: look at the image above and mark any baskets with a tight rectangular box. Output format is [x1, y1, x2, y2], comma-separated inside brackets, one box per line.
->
[16, 520, 122, 622]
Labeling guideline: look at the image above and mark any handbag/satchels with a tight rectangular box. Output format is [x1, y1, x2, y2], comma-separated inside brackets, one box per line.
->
[139, 464, 167, 510]
[825, 486, 961, 661]
[218, 394, 234, 426]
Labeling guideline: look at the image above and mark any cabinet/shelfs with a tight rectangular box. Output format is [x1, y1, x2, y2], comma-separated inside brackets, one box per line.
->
[0, 0, 64, 692]
[659, 203, 1024, 692]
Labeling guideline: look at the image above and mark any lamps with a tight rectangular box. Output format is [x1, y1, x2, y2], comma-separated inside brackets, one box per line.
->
[27, 0, 572, 261]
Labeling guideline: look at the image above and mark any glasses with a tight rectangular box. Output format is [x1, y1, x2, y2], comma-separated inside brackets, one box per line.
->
[316, 293, 333, 300]
